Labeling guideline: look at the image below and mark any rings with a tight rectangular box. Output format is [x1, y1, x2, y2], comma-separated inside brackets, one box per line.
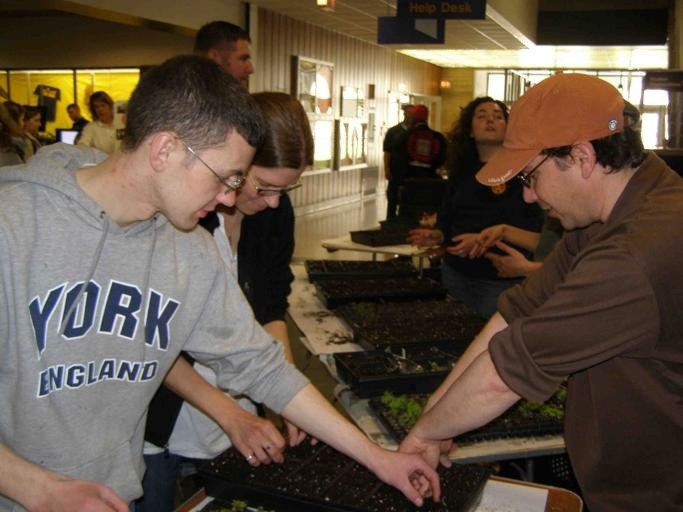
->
[264, 443, 274, 450]
[248, 456, 256, 465]
[246, 455, 253, 462]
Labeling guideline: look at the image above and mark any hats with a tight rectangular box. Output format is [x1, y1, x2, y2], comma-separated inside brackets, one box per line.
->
[474, 71, 625, 187]
[408, 104, 427, 120]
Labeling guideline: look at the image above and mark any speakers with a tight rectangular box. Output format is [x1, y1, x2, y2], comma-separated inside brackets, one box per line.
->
[377, 16, 445, 44]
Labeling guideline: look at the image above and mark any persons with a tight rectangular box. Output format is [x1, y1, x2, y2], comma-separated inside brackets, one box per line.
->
[396, 73, 683, 512]
[129, 93, 320, 512]
[193, 21, 255, 88]
[0, 91, 126, 167]
[0, 55, 441, 512]
[383, 90, 544, 269]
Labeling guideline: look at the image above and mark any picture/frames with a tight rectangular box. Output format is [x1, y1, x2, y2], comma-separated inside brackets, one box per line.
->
[294, 55, 336, 118]
[340, 85, 358, 118]
[335, 117, 368, 172]
[296, 115, 334, 176]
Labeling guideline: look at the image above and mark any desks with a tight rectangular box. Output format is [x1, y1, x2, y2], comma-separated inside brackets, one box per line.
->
[320, 225, 446, 276]
[173, 473, 583, 512]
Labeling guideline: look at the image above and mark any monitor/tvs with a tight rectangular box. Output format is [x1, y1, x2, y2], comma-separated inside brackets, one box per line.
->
[56, 127, 80, 145]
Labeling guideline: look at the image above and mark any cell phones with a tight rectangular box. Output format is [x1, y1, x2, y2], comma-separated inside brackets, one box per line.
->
[439, 238, 462, 247]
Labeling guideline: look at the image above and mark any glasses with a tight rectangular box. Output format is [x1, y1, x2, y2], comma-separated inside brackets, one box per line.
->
[247, 171, 302, 196]
[181, 140, 245, 194]
[516, 152, 549, 188]
